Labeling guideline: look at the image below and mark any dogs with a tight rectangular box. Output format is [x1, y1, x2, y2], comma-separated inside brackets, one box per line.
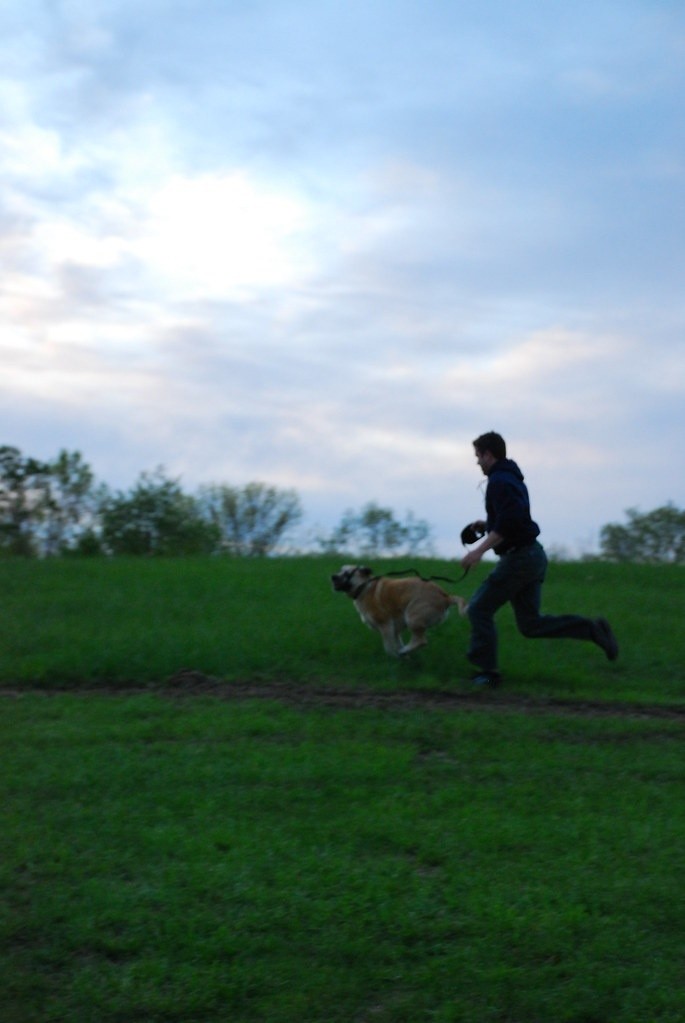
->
[331, 563, 473, 659]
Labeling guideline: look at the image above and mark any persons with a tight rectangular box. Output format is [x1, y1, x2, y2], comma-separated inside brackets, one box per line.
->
[461, 432, 618, 689]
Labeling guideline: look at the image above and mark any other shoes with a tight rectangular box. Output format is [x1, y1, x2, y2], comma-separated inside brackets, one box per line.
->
[470, 670, 499, 690]
[594, 618, 618, 661]
